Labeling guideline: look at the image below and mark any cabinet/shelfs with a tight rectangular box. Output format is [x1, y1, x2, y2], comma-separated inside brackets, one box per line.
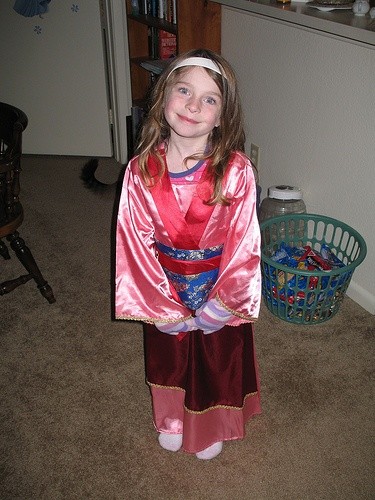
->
[125, 0, 222, 113]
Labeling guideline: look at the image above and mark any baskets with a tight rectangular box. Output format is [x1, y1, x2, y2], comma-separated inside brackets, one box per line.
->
[259, 213, 368, 324]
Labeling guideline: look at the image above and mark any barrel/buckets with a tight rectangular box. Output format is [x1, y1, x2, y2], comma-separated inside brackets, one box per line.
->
[259, 184, 307, 246]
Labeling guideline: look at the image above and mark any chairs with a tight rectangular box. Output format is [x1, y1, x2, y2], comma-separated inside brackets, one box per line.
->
[0, 102, 57, 305]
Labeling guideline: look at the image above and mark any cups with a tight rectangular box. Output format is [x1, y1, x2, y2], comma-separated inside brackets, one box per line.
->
[352, 0, 370, 16]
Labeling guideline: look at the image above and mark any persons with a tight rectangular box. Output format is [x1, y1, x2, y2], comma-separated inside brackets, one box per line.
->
[115, 48, 261, 460]
